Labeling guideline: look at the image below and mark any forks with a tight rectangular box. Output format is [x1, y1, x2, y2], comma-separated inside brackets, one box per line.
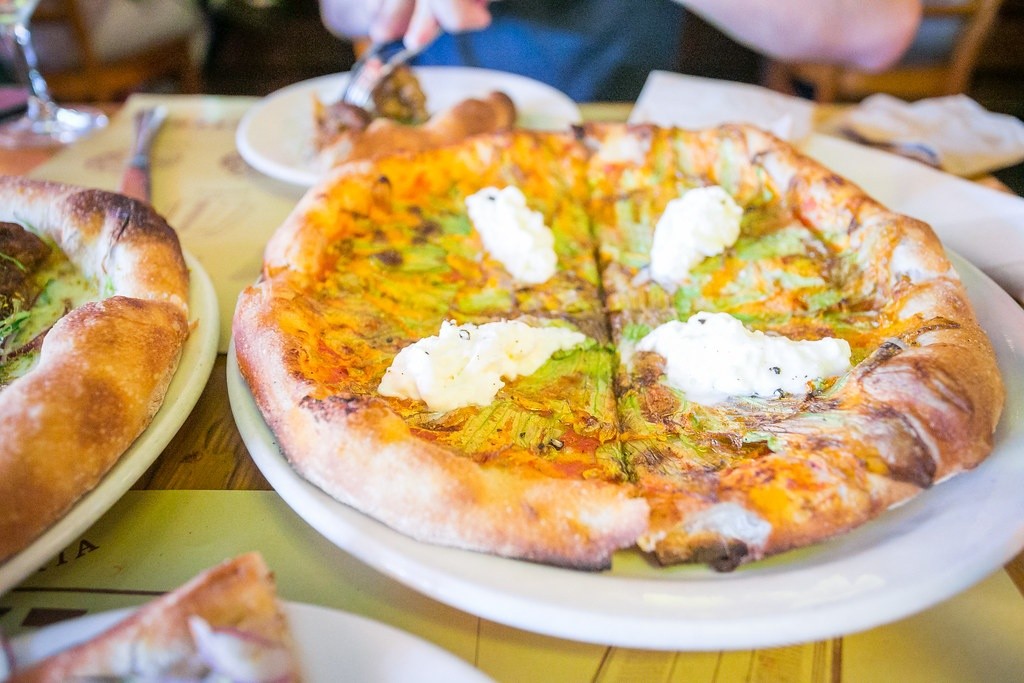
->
[339, 35, 425, 107]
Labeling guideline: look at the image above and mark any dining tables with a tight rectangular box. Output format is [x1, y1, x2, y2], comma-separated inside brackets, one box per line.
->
[0, 105, 1023, 683]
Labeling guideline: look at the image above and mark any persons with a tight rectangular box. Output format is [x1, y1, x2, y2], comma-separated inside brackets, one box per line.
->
[316, 1, 923, 106]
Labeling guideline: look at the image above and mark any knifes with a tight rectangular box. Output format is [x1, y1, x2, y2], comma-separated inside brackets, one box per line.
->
[116, 103, 170, 206]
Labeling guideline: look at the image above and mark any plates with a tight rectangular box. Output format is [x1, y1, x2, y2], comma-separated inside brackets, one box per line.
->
[0, 244, 220, 598]
[235, 66, 585, 187]
[226, 243, 1023, 653]
[0, 599, 493, 683]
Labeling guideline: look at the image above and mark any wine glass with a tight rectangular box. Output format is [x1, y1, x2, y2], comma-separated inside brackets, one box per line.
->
[0, 0, 110, 148]
